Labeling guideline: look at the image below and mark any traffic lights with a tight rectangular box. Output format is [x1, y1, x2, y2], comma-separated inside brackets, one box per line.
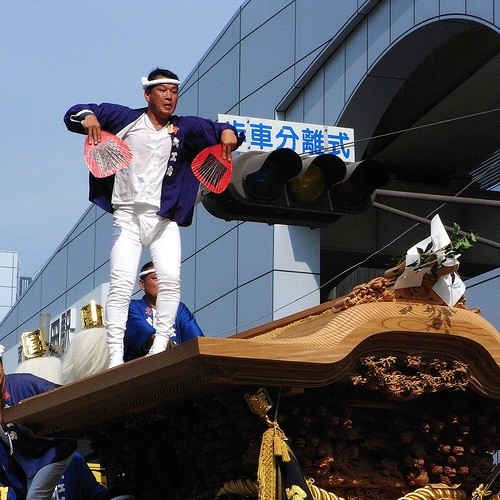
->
[200, 147, 378, 228]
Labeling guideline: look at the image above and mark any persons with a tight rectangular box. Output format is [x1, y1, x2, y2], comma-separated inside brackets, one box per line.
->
[64, 68, 245, 369]
[123, 261, 206, 362]
[0, 345, 78, 500]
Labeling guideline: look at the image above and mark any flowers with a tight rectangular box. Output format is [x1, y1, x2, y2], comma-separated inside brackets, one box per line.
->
[385, 214, 478, 307]
